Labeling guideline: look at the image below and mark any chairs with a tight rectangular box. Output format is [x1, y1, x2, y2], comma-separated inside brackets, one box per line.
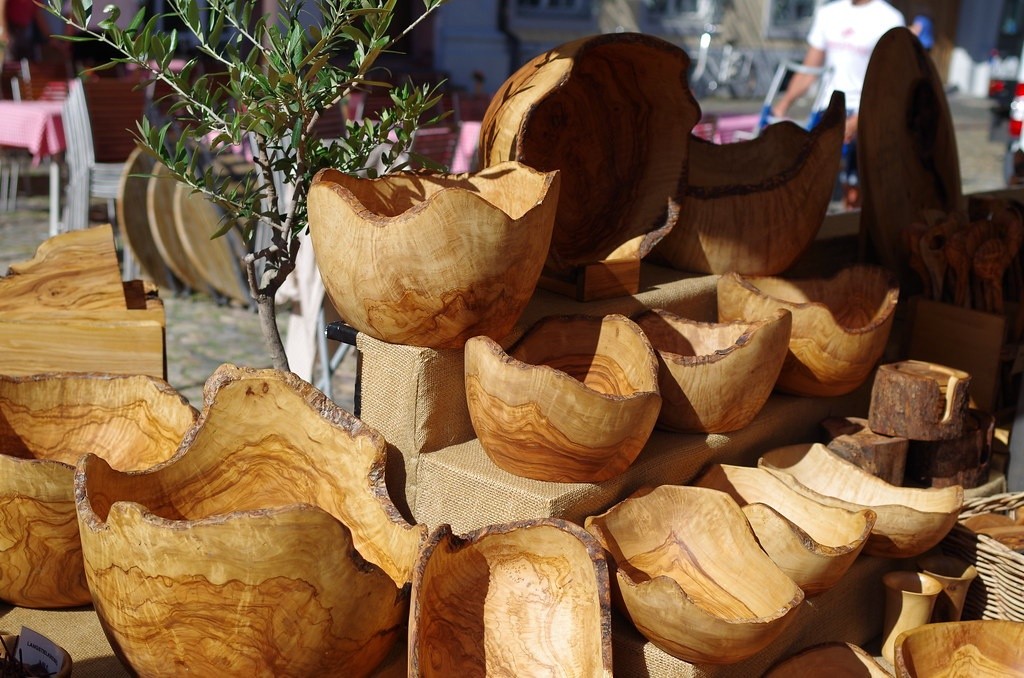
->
[6, 54, 230, 281]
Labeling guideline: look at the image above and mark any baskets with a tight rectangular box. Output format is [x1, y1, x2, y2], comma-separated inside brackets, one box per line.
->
[941, 492, 1024, 623]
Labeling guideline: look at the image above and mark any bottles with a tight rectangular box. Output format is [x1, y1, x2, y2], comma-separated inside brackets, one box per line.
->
[985, 47, 1024, 187]
[879, 555, 978, 667]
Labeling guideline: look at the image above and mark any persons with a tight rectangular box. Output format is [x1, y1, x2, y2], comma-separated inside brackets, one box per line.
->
[771, 0, 932, 211]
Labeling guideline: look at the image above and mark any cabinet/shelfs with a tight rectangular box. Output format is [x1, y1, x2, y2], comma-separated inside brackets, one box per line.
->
[322, 256, 883, 542]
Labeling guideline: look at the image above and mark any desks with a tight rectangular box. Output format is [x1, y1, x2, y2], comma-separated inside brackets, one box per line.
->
[0, 98, 69, 237]
